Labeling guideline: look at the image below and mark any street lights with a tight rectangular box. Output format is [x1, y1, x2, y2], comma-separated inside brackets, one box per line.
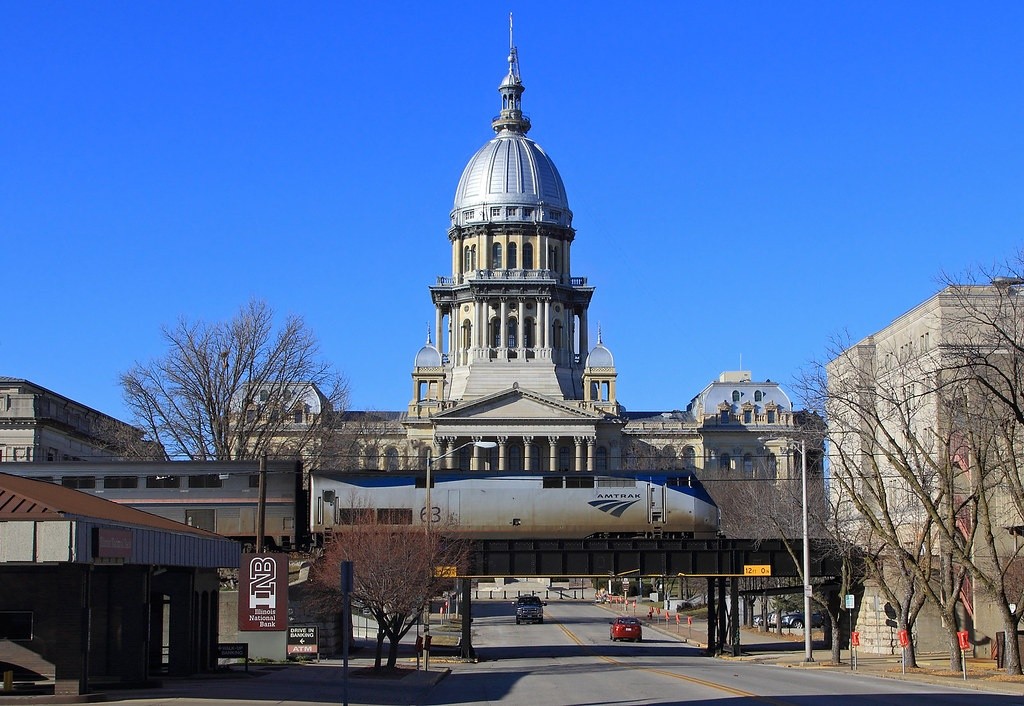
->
[756, 435, 814, 662]
[423, 440, 499, 671]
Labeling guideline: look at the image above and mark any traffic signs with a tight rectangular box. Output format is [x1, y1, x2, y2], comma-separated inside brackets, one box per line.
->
[287, 625, 319, 655]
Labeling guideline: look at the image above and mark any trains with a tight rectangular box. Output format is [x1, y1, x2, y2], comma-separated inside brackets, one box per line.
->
[0, 459, 723, 554]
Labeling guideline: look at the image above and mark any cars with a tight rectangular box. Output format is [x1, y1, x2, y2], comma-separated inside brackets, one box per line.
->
[751, 608, 823, 629]
[609, 616, 642, 642]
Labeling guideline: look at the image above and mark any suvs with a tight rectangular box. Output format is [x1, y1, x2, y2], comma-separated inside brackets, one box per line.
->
[511, 595, 547, 624]
